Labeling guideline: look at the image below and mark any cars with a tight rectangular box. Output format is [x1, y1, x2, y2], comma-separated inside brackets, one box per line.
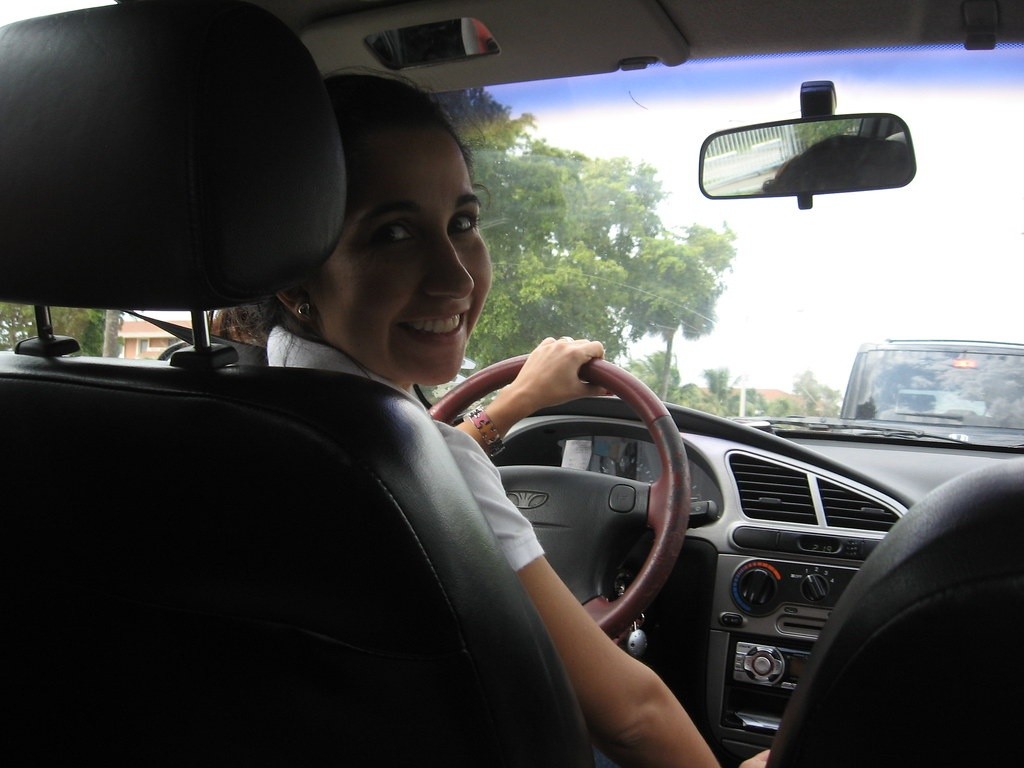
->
[840, 338, 1024, 437]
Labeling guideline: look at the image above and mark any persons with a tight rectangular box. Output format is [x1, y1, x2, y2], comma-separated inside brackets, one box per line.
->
[210, 65, 769, 768]
[763, 131, 911, 195]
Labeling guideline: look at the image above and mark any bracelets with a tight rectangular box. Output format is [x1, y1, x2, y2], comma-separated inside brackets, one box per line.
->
[463, 405, 505, 457]
